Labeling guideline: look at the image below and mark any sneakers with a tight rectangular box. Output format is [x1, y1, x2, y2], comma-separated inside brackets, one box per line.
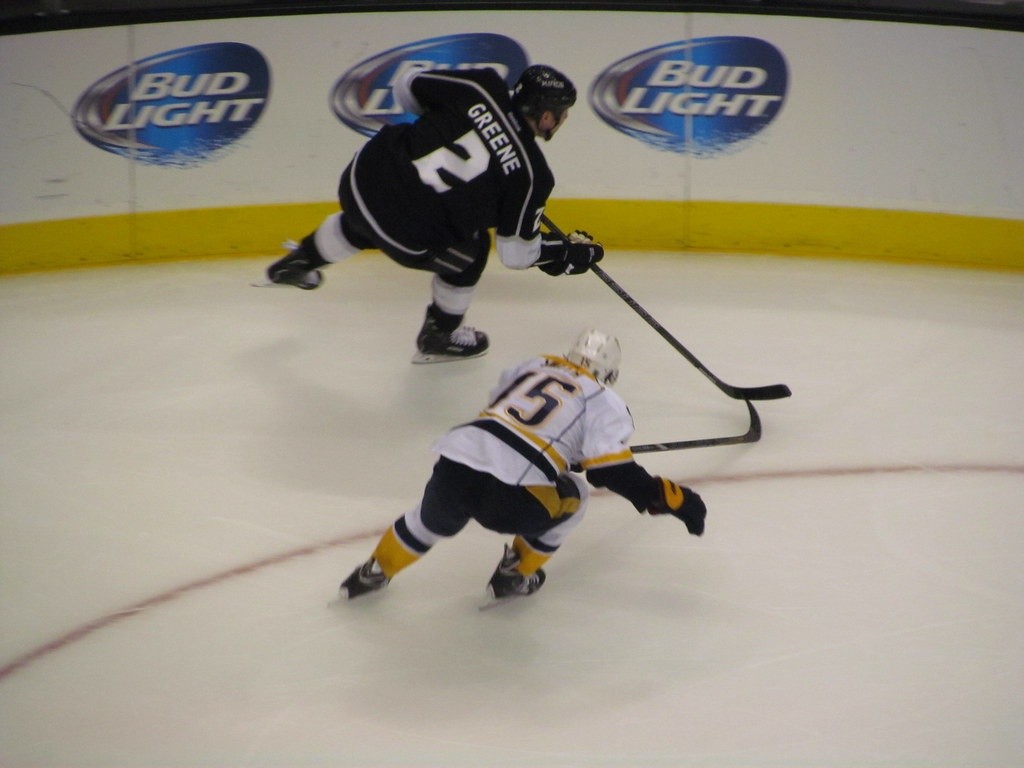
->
[477, 543, 546, 610]
[410, 302, 490, 364]
[326, 557, 392, 607]
[250, 231, 333, 291]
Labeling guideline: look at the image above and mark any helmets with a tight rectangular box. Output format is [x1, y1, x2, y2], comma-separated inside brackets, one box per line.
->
[567, 329, 623, 387]
[511, 65, 577, 122]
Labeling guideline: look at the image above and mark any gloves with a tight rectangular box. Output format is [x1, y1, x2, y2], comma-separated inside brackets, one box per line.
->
[538, 230, 604, 278]
[647, 476, 707, 537]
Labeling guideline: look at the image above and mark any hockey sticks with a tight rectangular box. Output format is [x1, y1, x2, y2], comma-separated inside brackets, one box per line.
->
[629, 398, 763, 458]
[539, 211, 791, 398]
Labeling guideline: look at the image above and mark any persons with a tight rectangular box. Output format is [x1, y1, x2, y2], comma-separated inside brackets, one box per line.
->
[333, 320, 710, 604]
[263, 58, 605, 361]
